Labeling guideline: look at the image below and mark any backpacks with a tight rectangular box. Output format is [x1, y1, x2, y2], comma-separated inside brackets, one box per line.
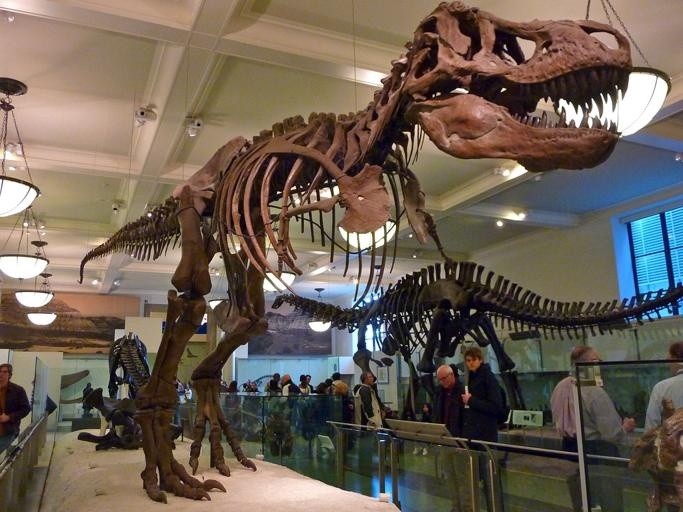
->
[483, 372, 510, 423]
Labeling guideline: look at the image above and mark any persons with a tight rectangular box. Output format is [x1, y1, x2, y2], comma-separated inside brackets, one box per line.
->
[437, 364, 472, 447]
[83, 383, 93, 415]
[461, 347, 508, 512]
[644, 342, 683, 433]
[550, 348, 637, 512]
[171, 372, 432, 467]
[0, 364, 31, 455]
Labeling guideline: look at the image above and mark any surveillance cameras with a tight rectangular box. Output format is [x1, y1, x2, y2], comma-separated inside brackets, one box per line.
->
[112, 203, 119, 211]
[185, 117, 203, 136]
[135, 106, 157, 127]
